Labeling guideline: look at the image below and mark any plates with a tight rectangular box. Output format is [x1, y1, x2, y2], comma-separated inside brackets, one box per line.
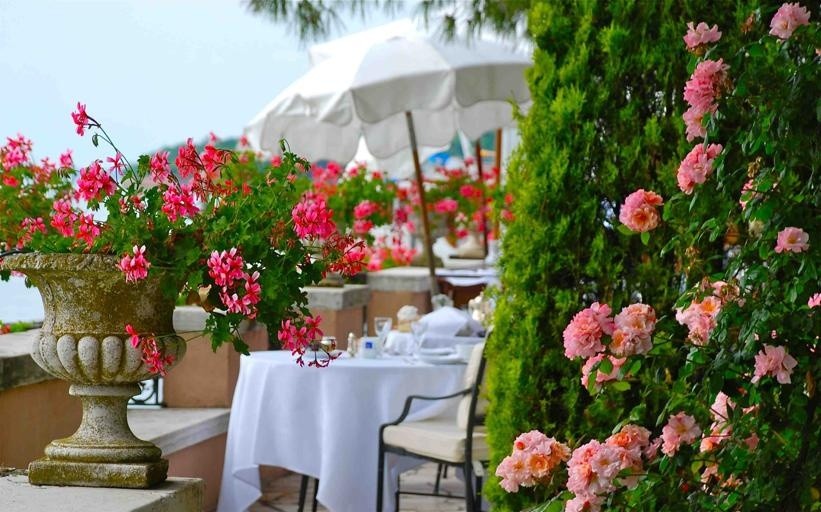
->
[415, 347, 458, 364]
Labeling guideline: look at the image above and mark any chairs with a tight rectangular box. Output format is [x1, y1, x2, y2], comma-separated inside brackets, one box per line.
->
[378, 325, 496, 512]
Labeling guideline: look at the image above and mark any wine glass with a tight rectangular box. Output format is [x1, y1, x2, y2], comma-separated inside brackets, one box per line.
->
[411, 320, 428, 347]
[375, 317, 392, 359]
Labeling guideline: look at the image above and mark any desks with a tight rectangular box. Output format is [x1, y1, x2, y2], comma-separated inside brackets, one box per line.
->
[385, 281, 496, 348]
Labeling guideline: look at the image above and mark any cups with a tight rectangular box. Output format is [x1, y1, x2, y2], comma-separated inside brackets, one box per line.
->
[455, 343, 477, 363]
[358, 337, 379, 358]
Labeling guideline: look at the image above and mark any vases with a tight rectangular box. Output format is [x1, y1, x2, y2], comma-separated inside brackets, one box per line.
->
[0, 254, 187, 487]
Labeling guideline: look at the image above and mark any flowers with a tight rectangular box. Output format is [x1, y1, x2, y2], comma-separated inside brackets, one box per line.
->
[616, 3, 817, 275]
[0, 101, 512, 377]
[495, 276, 820, 511]
